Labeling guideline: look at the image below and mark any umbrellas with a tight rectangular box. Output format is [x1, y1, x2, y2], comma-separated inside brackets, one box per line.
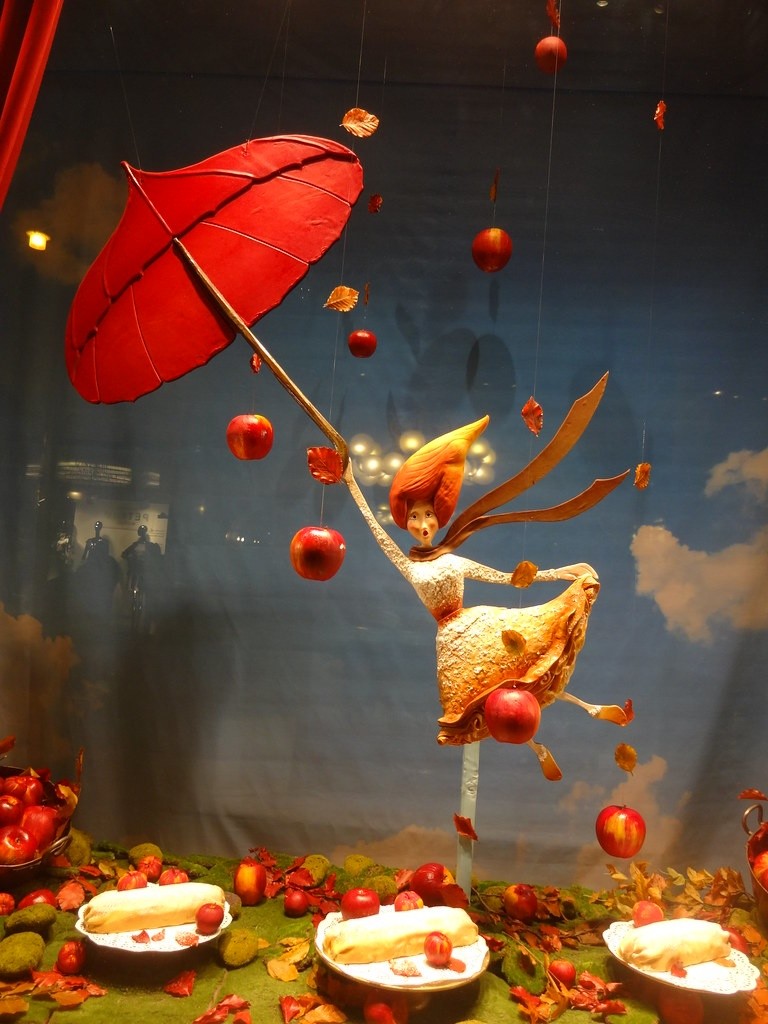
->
[65, 134, 364, 478]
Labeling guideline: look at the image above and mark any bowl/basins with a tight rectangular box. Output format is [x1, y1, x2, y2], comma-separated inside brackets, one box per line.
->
[2, 766, 73, 877]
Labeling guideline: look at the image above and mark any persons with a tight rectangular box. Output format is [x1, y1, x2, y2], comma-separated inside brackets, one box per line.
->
[121, 525, 158, 623]
[82, 521, 110, 563]
[334, 414, 626, 780]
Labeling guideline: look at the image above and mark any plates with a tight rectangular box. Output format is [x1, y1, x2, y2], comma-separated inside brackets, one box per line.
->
[316, 906, 491, 993]
[603, 915, 760, 995]
[74, 883, 232, 963]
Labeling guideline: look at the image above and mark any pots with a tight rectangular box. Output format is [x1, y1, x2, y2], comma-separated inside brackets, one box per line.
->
[742, 804, 768, 916]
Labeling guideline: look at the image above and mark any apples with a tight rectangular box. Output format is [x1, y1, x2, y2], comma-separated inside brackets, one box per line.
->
[0, 773, 768, 1024]
[290, 527, 346, 582]
[348, 330, 376, 358]
[485, 687, 541, 744]
[472, 228, 512, 273]
[535, 36, 567, 73]
[226, 414, 273, 460]
